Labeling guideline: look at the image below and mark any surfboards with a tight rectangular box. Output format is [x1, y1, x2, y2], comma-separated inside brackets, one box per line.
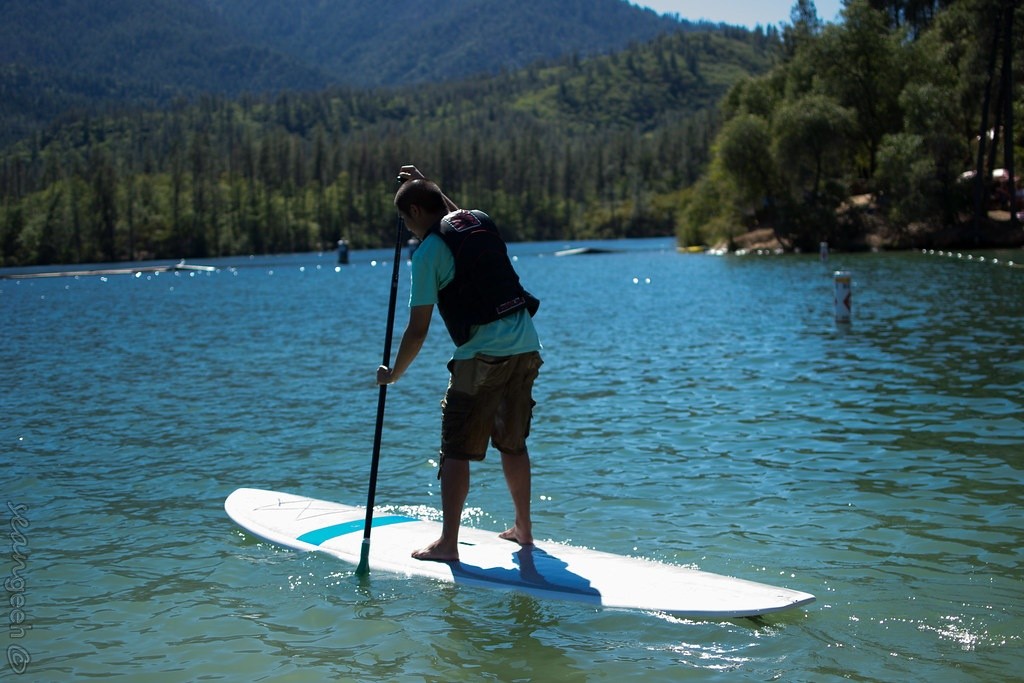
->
[224, 487, 815, 617]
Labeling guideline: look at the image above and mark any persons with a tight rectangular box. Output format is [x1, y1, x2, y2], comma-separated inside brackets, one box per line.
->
[376, 165, 543, 560]
[338, 235, 349, 263]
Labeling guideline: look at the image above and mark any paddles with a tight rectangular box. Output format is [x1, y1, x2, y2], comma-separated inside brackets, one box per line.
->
[355, 177, 407, 573]
